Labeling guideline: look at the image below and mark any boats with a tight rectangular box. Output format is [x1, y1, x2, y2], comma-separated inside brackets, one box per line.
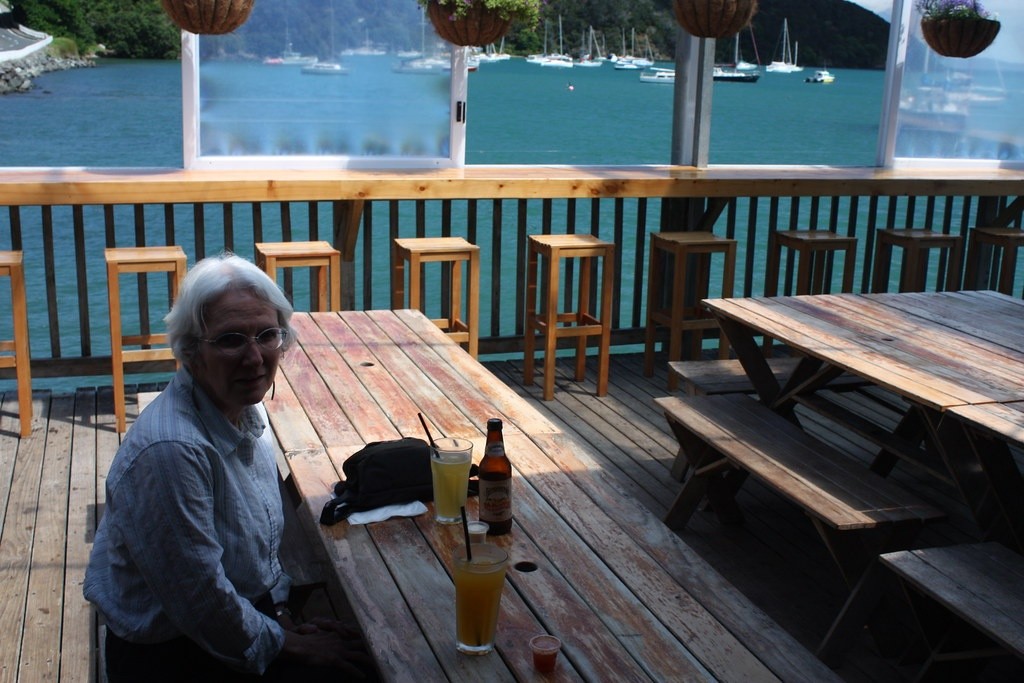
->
[803, 69, 835, 83]
[263, 22, 319, 65]
[939, 72, 1005, 105]
[446, 36, 510, 73]
[898, 47, 970, 132]
[397, 49, 423, 58]
[736, 60, 758, 71]
[342, 29, 386, 56]
[641, 71, 675, 84]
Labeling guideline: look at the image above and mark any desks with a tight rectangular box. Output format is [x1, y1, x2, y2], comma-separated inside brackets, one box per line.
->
[697, 290, 1023, 448]
[262, 310, 848, 682]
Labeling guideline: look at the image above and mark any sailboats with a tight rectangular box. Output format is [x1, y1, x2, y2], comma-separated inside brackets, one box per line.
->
[713, 23, 760, 83]
[393, 6, 446, 75]
[302, 3, 350, 73]
[765, 17, 804, 74]
[527, 13, 655, 69]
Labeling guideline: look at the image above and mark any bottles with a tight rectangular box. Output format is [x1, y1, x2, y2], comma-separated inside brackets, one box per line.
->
[479, 418, 512, 535]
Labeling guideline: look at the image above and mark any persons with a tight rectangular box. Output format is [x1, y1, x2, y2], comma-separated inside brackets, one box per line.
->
[83, 247, 371, 683]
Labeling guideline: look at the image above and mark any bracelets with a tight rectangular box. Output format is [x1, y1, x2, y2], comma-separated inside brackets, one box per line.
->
[272, 605, 290, 619]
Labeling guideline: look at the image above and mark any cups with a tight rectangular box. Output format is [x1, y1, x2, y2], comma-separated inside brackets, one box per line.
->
[466, 522, 489, 543]
[529, 636, 560, 672]
[452, 542, 508, 655]
[430, 438, 473, 524]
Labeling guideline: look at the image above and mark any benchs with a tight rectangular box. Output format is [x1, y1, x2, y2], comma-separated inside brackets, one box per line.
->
[655, 356, 1024, 683]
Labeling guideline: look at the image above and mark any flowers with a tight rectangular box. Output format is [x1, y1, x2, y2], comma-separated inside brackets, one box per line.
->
[915, 0, 992, 21]
[415, 0, 549, 33]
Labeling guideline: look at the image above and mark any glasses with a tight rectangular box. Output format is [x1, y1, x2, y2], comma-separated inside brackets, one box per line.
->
[194, 328, 289, 356]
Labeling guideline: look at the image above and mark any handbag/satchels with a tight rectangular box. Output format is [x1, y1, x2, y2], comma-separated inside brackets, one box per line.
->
[317, 438, 481, 526]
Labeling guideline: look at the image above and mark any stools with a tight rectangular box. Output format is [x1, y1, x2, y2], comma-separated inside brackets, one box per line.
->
[107, 246, 183, 433]
[762, 230, 857, 356]
[643, 230, 735, 390]
[964, 228, 1023, 296]
[524, 234, 612, 401]
[0, 252, 33, 441]
[872, 227, 962, 295]
[391, 237, 480, 361]
[256, 241, 341, 315]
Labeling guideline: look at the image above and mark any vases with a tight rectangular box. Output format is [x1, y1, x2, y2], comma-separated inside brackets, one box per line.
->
[920, 18, 1001, 57]
[673, 0, 753, 39]
[161, 0, 258, 34]
[428, 3, 512, 47]
